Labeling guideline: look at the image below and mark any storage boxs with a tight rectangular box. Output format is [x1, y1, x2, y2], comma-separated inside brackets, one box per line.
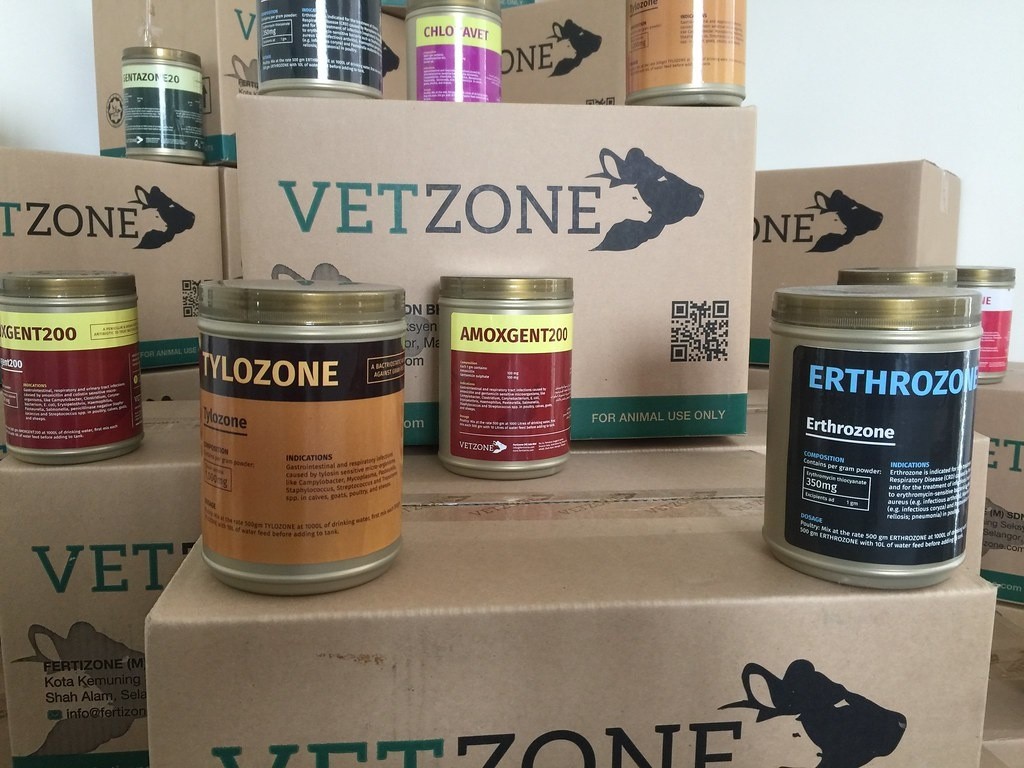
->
[1, 0, 1024, 768]
